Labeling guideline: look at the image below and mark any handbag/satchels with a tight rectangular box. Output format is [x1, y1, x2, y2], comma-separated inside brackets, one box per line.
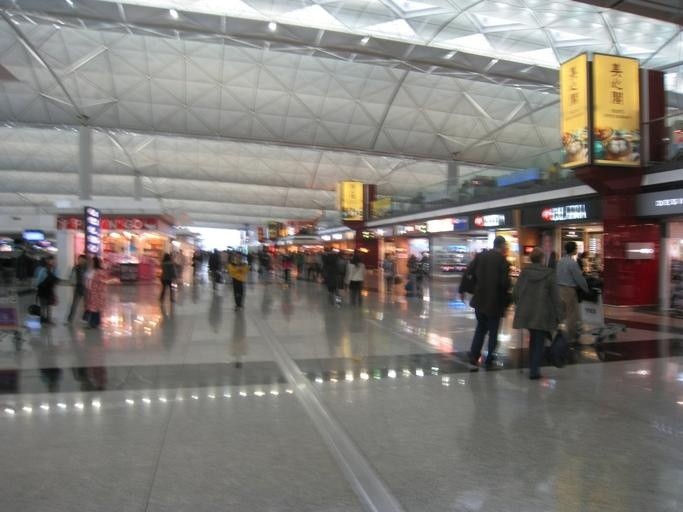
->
[459, 255, 479, 294]
[544, 329, 568, 370]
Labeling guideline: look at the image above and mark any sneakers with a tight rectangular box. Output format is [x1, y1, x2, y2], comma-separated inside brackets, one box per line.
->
[465, 355, 544, 381]
[37, 314, 105, 332]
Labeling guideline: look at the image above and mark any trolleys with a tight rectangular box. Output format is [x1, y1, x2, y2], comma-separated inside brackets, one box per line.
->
[0, 288, 29, 351]
[574, 287, 628, 360]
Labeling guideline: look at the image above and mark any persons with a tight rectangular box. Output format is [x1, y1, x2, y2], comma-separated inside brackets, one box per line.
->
[556, 348, 613, 483]
[381, 250, 397, 301]
[207, 295, 224, 333]
[227, 311, 250, 368]
[458, 235, 512, 372]
[408, 263, 431, 294]
[66, 324, 94, 396]
[83, 256, 106, 330]
[465, 369, 510, 498]
[512, 249, 566, 379]
[32, 252, 65, 325]
[157, 251, 176, 304]
[381, 300, 397, 327]
[172, 243, 368, 312]
[553, 240, 596, 348]
[513, 378, 559, 498]
[34, 323, 63, 394]
[548, 251, 557, 270]
[158, 303, 177, 355]
[348, 308, 363, 354]
[61, 253, 89, 325]
[78, 325, 108, 396]
[323, 307, 345, 360]
[408, 293, 428, 318]
